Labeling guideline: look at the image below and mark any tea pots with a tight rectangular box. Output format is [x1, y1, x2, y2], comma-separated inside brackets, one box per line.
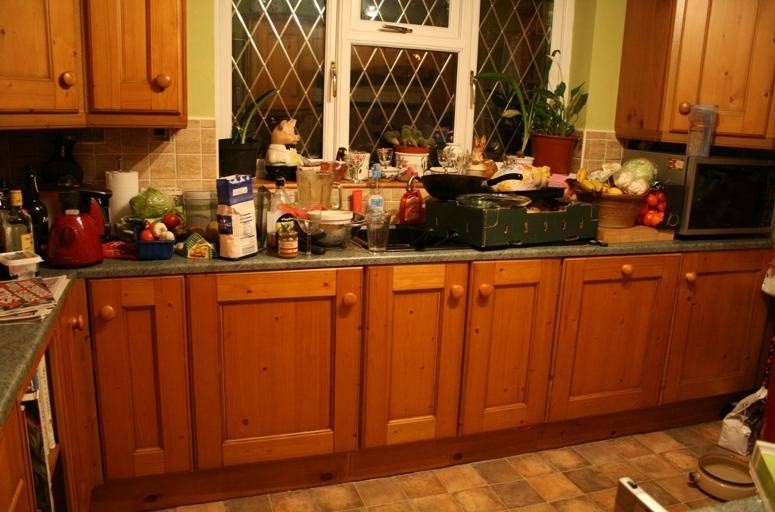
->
[50, 198, 105, 269]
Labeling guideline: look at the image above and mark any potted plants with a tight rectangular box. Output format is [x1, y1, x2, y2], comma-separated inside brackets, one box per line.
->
[383, 125, 436, 176]
[528, 47, 588, 176]
[474, 49, 562, 166]
[219, 88, 278, 179]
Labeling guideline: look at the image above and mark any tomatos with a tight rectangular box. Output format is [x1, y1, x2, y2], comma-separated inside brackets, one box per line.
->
[139, 229, 156, 241]
[162, 213, 179, 228]
[637, 191, 668, 227]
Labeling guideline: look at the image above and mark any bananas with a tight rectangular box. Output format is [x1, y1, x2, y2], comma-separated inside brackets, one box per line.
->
[576, 167, 607, 192]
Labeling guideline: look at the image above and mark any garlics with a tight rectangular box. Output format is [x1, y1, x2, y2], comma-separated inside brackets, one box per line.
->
[152, 223, 174, 240]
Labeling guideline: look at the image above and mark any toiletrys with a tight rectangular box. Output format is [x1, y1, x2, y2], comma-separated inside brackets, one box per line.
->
[368, 193, 383, 221]
[399, 189, 422, 225]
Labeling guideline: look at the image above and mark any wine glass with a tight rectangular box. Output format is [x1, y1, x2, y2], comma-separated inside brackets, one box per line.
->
[376, 148, 393, 182]
[437, 150, 451, 174]
[349, 149, 365, 184]
[298, 202, 322, 260]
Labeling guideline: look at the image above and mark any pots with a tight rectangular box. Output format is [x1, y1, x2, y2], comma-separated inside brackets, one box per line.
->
[418, 173, 523, 200]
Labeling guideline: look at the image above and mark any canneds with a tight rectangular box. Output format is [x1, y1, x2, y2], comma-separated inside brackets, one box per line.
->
[278, 231, 299, 258]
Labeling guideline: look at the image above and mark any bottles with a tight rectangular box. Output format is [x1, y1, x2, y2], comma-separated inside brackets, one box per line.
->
[366, 164, 384, 213]
[266, 178, 298, 258]
[1, 165, 49, 267]
[684, 104, 715, 158]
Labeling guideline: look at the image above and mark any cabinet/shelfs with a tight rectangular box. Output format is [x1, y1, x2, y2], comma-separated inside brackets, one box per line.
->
[615, 0, 775, 150]
[358, 258, 563, 482]
[0, 0, 188, 130]
[0, 403, 38, 512]
[188, 268, 366, 502]
[547, 253, 684, 450]
[659, 247, 775, 429]
[48, 277, 195, 512]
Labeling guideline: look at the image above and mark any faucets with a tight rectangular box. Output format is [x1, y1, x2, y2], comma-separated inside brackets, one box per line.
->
[407, 176, 422, 191]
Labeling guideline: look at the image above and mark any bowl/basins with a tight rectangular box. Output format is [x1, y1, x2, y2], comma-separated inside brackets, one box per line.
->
[430, 166, 458, 174]
[595, 193, 643, 230]
[382, 168, 401, 180]
[296, 210, 368, 251]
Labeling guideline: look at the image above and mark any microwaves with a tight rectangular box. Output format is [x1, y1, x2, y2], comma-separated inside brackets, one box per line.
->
[620, 146, 774, 240]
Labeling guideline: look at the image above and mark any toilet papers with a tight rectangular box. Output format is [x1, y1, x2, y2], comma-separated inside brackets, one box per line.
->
[106, 168, 140, 235]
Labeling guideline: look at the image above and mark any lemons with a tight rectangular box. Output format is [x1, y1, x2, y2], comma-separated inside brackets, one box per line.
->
[605, 188, 623, 195]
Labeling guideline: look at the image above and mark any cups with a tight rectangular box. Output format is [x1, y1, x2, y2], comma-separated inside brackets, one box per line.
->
[502, 153, 518, 171]
[182, 190, 217, 234]
[365, 213, 392, 251]
[297, 170, 345, 211]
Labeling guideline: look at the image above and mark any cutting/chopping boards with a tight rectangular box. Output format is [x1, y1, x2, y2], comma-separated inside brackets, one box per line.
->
[597, 226, 660, 244]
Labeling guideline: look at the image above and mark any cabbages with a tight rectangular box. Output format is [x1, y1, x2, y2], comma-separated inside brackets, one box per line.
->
[130, 188, 172, 220]
[622, 157, 656, 183]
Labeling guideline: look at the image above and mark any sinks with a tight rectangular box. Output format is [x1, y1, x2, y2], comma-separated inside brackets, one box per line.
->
[351, 229, 447, 250]
